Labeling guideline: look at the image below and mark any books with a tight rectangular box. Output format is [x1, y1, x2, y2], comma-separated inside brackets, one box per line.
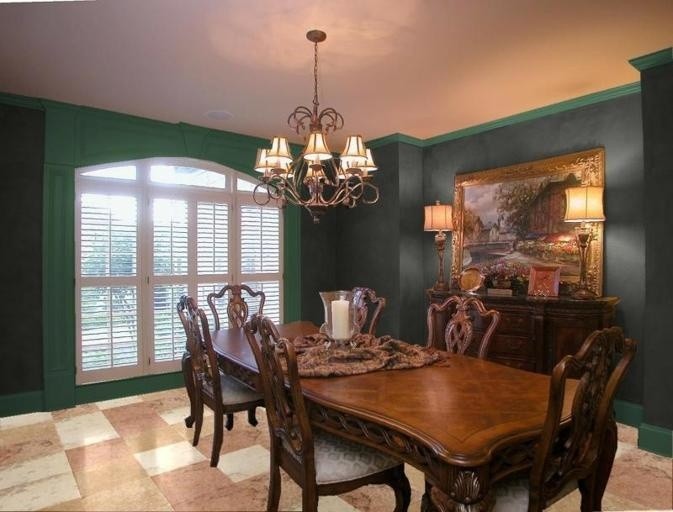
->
[487, 287, 518, 297]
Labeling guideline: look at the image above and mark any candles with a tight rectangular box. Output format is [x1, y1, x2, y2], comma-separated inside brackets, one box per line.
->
[331, 296, 349, 345]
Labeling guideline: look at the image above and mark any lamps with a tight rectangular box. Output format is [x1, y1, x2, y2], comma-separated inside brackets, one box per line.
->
[563, 183, 606, 300]
[253, 31, 380, 224]
[422, 200, 455, 294]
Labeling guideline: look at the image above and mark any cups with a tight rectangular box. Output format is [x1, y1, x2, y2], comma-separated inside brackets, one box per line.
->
[318, 289, 364, 343]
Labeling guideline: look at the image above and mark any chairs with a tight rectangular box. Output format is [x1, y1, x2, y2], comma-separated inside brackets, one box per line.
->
[206, 285, 266, 430]
[422, 325, 637, 510]
[425, 297, 499, 360]
[243, 313, 412, 511]
[350, 288, 386, 337]
[178, 295, 268, 467]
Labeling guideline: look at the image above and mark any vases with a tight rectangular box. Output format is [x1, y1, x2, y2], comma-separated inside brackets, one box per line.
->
[495, 277, 511, 287]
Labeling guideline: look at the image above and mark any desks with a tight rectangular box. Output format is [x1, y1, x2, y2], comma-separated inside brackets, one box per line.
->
[181, 320, 618, 509]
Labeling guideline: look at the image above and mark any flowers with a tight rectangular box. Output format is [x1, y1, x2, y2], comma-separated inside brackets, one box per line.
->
[479, 262, 531, 279]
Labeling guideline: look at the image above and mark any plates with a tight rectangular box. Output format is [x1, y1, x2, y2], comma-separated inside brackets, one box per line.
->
[457, 267, 484, 292]
[528, 266, 561, 298]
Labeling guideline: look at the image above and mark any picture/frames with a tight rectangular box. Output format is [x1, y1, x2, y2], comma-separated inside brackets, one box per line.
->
[527, 262, 564, 300]
[448, 148, 605, 298]
